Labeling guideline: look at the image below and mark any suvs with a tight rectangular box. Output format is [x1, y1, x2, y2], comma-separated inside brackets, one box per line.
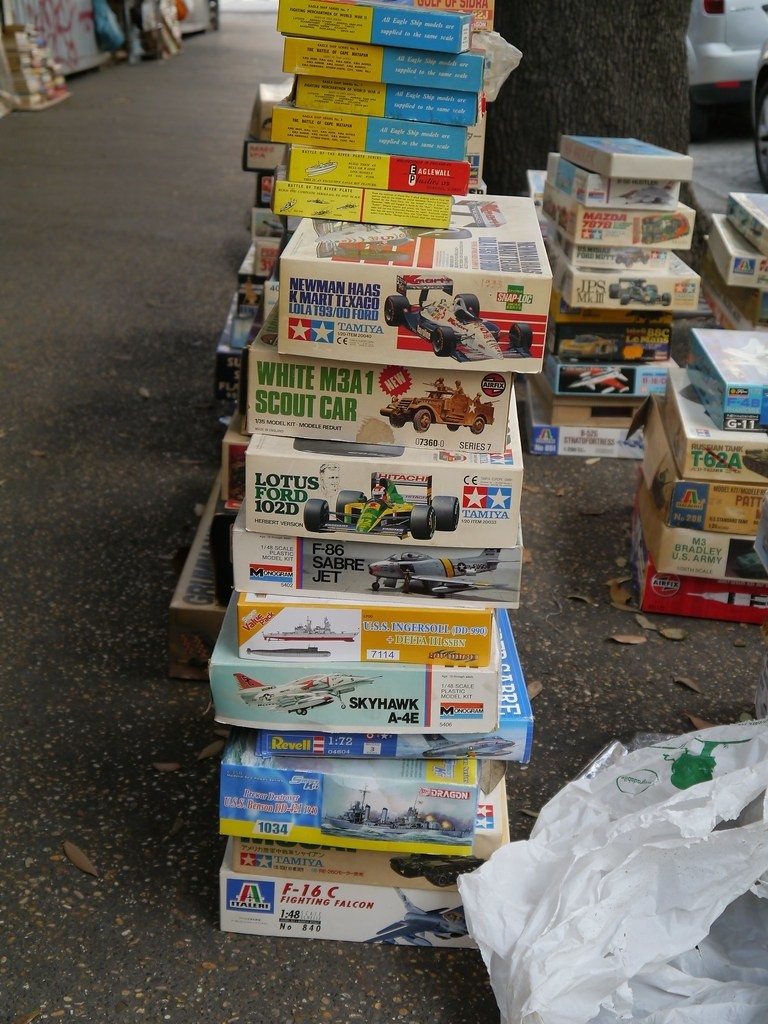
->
[683, 0, 768, 133]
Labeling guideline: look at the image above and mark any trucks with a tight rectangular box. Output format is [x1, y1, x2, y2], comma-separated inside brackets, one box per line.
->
[380, 376, 496, 435]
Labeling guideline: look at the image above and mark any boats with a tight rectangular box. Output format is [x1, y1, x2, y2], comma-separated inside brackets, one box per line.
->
[327, 784, 468, 844]
[260, 616, 358, 642]
[245, 644, 328, 656]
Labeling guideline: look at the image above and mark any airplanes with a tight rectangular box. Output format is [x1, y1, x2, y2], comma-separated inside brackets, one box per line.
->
[365, 890, 472, 945]
[623, 178, 678, 205]
[232, 669, 384, 716]
[354, 544, 505, 594]
[566, 366, 633, 393]
[418, 733, 517, 761]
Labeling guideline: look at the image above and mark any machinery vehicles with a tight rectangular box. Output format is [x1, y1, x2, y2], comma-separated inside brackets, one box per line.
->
[390, 854, 489, 890]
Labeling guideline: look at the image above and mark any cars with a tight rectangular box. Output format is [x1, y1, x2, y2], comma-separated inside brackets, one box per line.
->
[615, 248, 650, 267]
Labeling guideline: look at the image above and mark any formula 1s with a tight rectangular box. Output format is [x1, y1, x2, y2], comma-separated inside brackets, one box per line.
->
[313, 194, 508, 258]
[610, 277, 671, 305]
[561, 330, 618, 359]
[384, 269, 534, 365]
[298, 469, 466, 544]
[632, 211, 690, 243]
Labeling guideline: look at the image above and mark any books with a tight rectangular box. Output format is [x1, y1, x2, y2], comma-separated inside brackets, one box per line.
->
[5, 22, 68, 107]
